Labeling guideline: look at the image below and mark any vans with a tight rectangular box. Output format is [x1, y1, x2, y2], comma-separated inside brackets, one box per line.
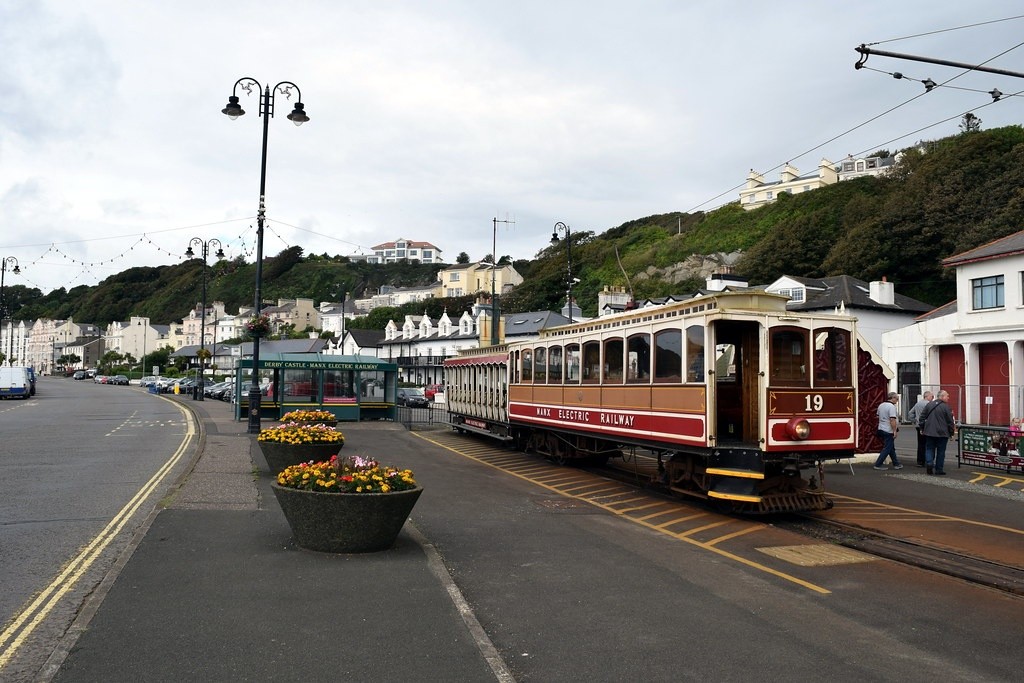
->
[0, 367, 37, 399]
[267, 381, 356, 397]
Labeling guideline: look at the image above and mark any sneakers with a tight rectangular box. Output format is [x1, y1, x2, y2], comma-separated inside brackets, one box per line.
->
[874, 466, 888, 470]
[894, 464, 903, 469]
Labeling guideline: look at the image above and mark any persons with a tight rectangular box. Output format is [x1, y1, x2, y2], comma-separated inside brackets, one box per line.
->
[874, 392, 903, 470]
[919, 390, 955, 475]
[1009, 417, 1024, 437]
[908, 391, 935, 468]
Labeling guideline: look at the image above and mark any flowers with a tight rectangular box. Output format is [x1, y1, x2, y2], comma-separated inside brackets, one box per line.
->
[242, 311, 273, 340]
[277, 455, 416, 493]
[257, 409, 345, 444]
[196, 349, 211, 359]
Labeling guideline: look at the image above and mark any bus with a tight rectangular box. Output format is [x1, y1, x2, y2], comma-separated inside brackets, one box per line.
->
[442, 290, 859, 514]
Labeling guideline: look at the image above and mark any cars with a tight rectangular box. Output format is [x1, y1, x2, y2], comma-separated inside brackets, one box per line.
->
[361, 377, 384, 388]
[87, 369, 97, 378]
[140, 376, 215, 394]
[396, 384, 444, 407]
[204, 381, 270, 403]
[73, 371, 89, 379]
[94, 375, 129, 385]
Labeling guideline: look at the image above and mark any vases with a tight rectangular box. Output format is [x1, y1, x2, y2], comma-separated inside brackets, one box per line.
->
[271, 480, 424, 553]
[258, 441, 345, 476]
[279, 420, 338, 427]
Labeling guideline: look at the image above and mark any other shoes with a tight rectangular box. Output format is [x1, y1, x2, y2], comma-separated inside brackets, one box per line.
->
[935, 472, 946, 475]
[917, 463, 923, 467]
[928, 472, 933, 475]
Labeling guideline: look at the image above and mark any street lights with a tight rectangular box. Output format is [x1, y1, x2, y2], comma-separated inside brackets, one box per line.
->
[49, 335, 59, 363]
[93, 324, 101, 360]
[221, 77, 309, 434]
[0, 256, 21, 330]
[549, 222, 572, 324]
[137, 317, 146, 377]
[60, 329, 72, 371]
[10, 293, 26, 367]
[184, 237, 225, 401]
[332, 280, 345, 355]
